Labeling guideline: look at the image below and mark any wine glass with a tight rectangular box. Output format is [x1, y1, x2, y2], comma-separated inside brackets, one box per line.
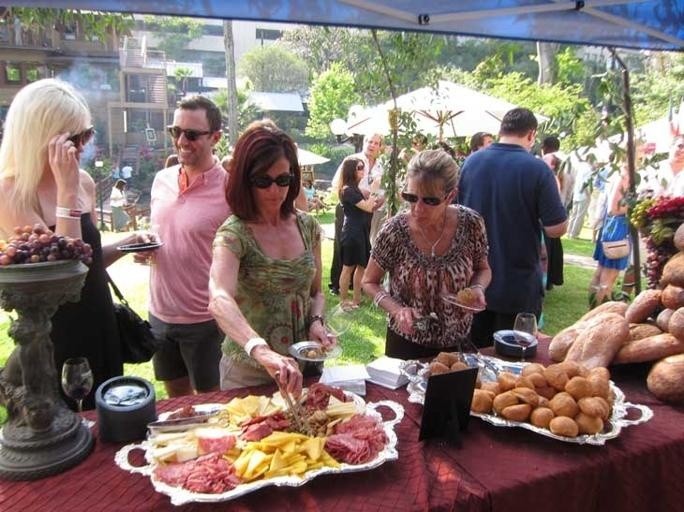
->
[61, 358, 94, 430]
[513, 312, 538, 375]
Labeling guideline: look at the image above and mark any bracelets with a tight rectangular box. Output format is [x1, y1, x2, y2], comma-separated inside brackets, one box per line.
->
[55, 207, 82, 219]
[207, 126, 336, 400]
[308, 314, 323, 326]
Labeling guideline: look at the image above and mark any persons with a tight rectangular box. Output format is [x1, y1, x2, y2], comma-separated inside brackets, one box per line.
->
[303, 131, 498, 310]
[112, 163, 121, 184]
[221, 156, 233, 172]
[360, 147, 492, 360]
[531, 134, 684, 308]
[449, 106, 567, 348]
[110, 179, 132, 233]
[121, 163, 134, 191]
[133, 95, 235, 399]
[244, 337, 267, 357]
[244, 118, 308, 211]
[0, 78, 161, 412]
[166, 155, 180, 168]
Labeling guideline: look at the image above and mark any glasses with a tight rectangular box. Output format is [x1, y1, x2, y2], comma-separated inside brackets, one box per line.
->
[59, 126, 96, 150]
[166, 123, 215, 142]
[249, 171, 300, 188]
[400, 182, 450, 207]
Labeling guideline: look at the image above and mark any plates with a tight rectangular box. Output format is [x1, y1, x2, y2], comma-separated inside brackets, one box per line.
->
[286, 339, 342, 363]
[493, 329, 538, 357]
[439, 293, 486, 313]
[114, 243, 163, 252]
[94, 375, 157, 440]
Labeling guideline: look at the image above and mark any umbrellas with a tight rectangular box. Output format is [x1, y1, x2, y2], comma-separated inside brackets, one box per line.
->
[331, 78, 551, 143]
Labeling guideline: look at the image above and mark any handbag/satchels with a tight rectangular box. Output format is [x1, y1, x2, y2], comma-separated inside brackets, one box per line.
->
[600, 239, 634, 261]
[104, 302, 159, 365]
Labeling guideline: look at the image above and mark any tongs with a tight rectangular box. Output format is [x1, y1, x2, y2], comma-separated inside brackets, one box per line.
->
[273, 371, 316, 435]
[454, 334, 499, 377]
[144, 408, 232, 430]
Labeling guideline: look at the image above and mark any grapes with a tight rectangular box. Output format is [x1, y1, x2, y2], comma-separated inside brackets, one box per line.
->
[630, 195, 684, 289]
[0, 224, 93, 265]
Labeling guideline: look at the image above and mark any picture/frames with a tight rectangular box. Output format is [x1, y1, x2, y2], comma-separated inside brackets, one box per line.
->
[419, 368, 481, 452]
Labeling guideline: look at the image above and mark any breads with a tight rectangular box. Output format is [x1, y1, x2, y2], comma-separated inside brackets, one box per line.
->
[427, 224, 684, 437]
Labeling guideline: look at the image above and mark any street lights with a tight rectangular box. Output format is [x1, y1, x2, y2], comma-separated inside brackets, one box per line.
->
[94, 155, 108, 231]
[330, 104, 366, 153]
